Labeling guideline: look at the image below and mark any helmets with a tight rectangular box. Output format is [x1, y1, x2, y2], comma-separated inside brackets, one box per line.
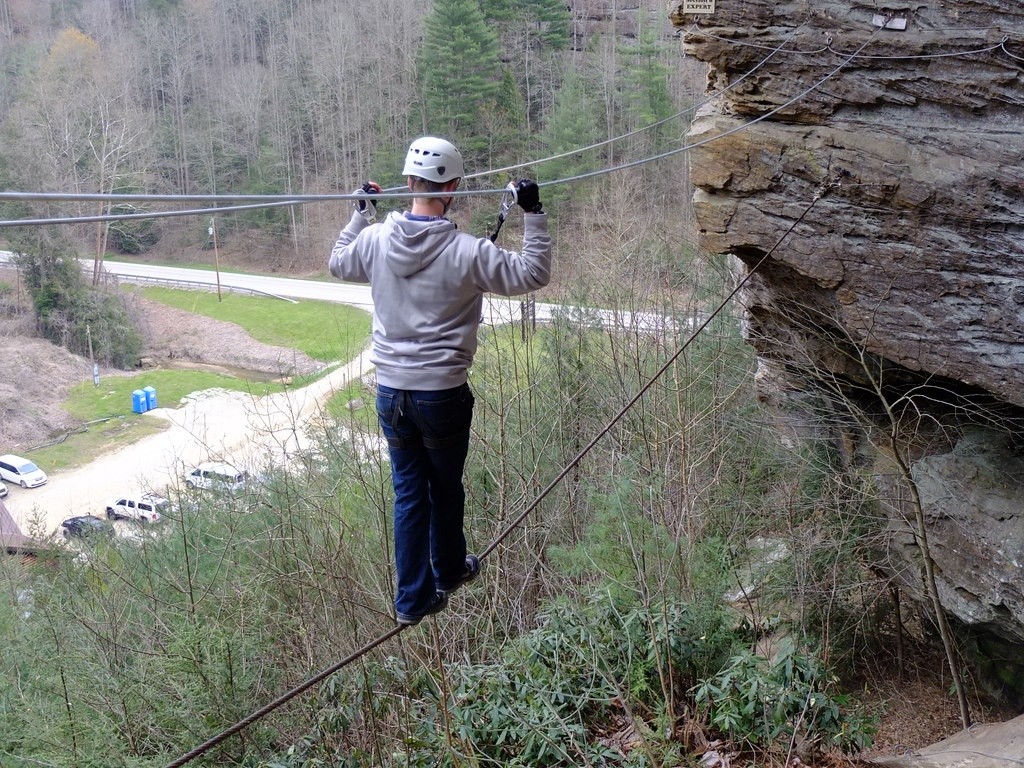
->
[402, 137, 465, 183]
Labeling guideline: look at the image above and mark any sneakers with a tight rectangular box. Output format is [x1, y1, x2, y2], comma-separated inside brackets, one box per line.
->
[435, 554, 481, 593]
[396, 590, 448, 624]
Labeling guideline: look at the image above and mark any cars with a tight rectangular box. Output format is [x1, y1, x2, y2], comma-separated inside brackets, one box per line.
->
[61, 515, 115, 548]
[0, 481, 9, 498]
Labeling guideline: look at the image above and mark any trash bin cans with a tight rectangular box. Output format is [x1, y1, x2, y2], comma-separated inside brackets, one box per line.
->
[132, 389, 148, 414]
[143, 385, 157, 410]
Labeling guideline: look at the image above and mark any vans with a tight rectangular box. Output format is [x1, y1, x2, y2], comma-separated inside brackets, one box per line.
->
[0, 454, 48, 488]
[185, 462, 250, 497]
[103, 491, 171, 528]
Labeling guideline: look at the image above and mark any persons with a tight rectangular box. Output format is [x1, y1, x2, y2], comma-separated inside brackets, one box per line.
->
[328, 137, 552, 624]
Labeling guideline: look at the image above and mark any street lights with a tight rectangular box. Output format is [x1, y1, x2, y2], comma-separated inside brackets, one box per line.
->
[208, 219, 221, 301]
